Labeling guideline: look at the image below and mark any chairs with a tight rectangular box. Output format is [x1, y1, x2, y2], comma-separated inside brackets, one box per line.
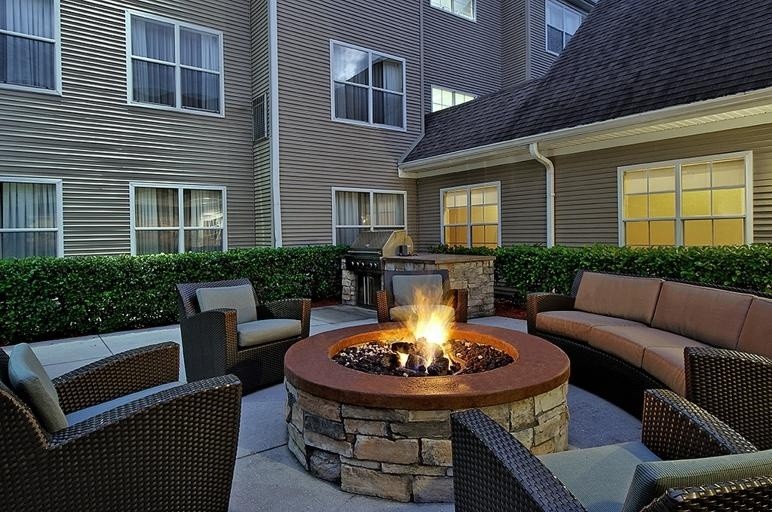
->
[451, 388, 772, 512]
[376, 268, 468, 324]
[1, 342, 243, 511]
[173, 278, 312, 397]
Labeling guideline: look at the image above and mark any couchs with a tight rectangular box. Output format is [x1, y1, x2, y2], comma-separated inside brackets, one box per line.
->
[526, 271, 771, 448]
